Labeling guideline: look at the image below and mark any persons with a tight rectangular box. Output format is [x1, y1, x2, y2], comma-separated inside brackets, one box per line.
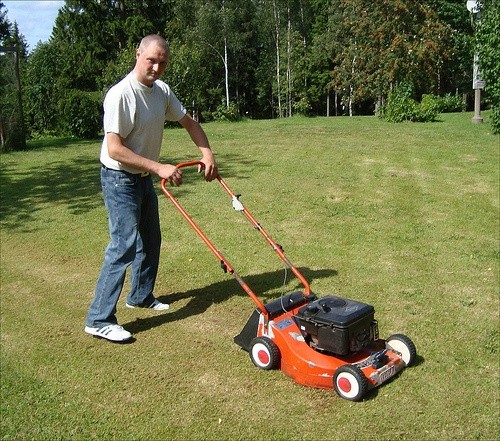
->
[84, 34, 219, 343]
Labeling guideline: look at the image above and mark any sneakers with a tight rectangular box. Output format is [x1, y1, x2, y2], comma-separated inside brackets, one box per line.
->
[84, 323, 132, 343]
[126, 298, 170, 310]
